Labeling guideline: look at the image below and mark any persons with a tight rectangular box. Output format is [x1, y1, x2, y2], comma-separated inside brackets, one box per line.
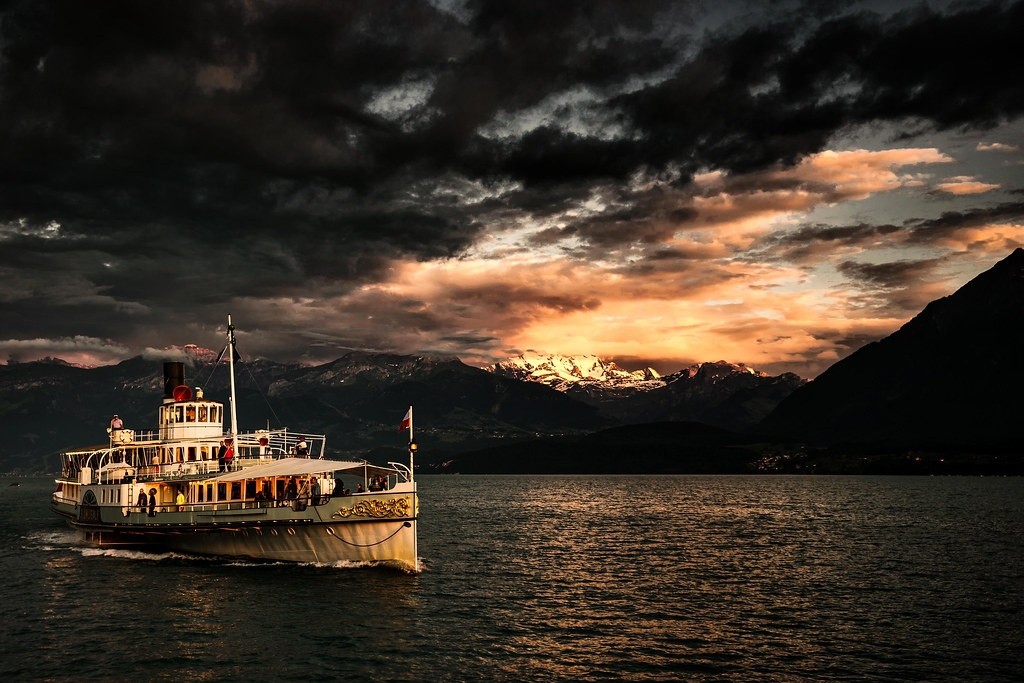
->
[148, 490, 155, 516]
[330, 471, 389, 493]
[295, 436, 307, 455]
[111, 415, 124, 448]
[217, 441, 235, 473]
[136, 488, 147, 513]
[253, 478, 321, 509]
[176, 489, 185, 511]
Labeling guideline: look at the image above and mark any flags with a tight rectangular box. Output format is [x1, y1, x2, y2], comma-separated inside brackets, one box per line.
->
[398, 405, 410, 434]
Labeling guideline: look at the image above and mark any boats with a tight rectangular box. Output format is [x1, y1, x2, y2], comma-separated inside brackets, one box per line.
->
[50, 312, 419, 571]
